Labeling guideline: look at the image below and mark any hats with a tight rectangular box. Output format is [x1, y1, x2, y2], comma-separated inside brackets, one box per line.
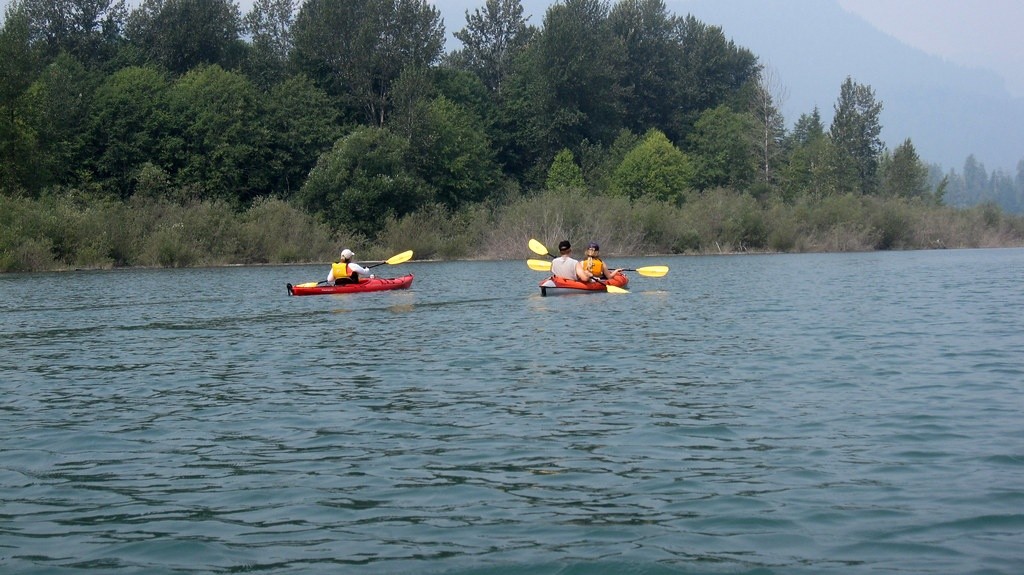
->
[341, 249, 355, 259]
[588, 243, 599, 250]
[559, 240, 571, 251]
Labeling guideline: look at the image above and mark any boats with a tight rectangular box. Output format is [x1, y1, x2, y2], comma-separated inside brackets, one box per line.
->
[539, 269, 630, 297]
[285, 272, 415, 297]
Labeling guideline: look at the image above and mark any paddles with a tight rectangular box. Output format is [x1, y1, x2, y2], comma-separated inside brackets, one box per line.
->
[297, 250, 413, 288]
[527, 258, 669, 278]
[528, 238, 629, 293]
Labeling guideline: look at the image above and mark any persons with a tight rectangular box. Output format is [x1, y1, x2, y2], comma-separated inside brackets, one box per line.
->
[327, 249, 370, 286]
[550, 240, 593, 282]
[579, 242, 624, 281]
